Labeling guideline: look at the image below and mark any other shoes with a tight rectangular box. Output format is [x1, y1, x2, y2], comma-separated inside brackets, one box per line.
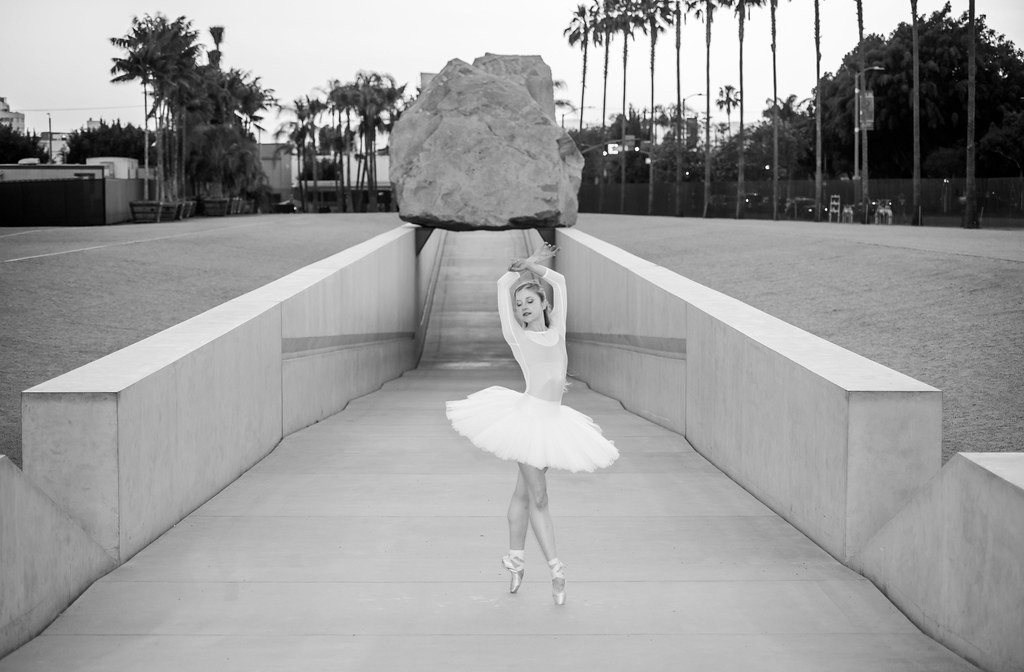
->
[501, 553, 526, 595]
[547, 557, 567, 606]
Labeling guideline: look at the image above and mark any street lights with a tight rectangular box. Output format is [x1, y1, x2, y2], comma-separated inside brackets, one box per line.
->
[853, 65, 886, 203]
[682, 93, 703, 144]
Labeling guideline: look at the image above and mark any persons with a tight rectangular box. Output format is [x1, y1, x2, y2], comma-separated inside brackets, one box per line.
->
[445, 242, 619, 605]
